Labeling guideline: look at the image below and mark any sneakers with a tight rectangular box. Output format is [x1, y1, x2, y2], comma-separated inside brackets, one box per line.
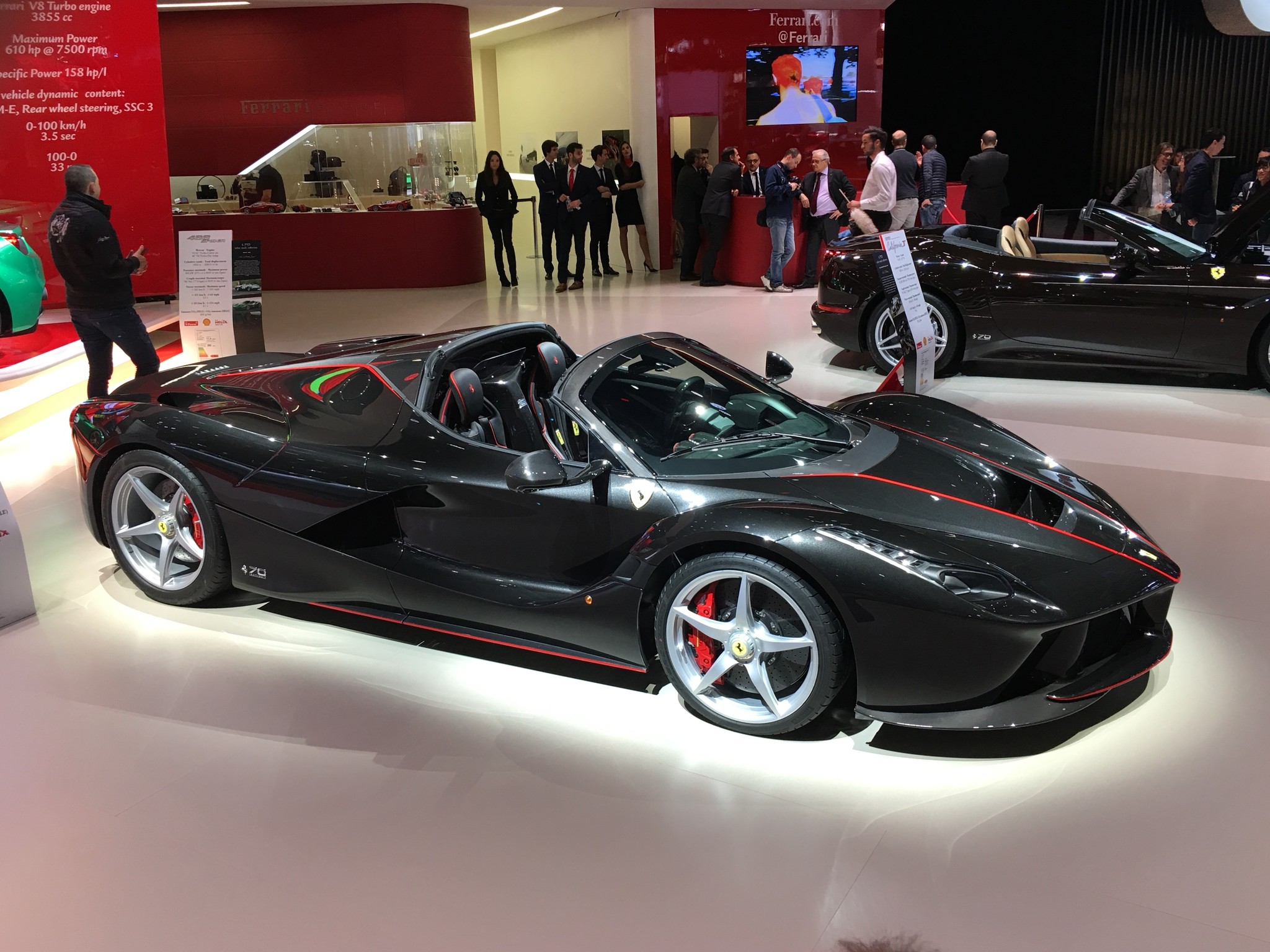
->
[760, 275, 773, 292]
[771, 283, 792, 292]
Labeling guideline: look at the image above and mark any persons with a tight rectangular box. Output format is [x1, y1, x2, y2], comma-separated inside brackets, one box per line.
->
[886, 129, 919, 232]
[960, 130, 1009, 229]
[847, 126, 897, 236]
[533, 139, 576, 280]
[475, 149, 519, 287]
[754, 54, 838, 122]
[699, 145, 745, 287]
[915, 134, 947, 226]
[802, 77, 836, 122]
[46, 165, 161, 399]
[550, 142, 598, 293]
[760, 148, 803, 292]
[1110, 127, 1269, 246]
[793, 149, 857, 289]
[740, 150, 771, 195]
[670, 149, 686, 259]
[672, 148, 709, 281]
[257, 164, 287, 212]
[614, 140, 660, 272]
[589, 145, 619, 277]
[231, 172, 259, 207]
[696, 147, 714, 188]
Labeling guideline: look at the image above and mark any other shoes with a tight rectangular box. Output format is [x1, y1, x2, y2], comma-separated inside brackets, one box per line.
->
[545, 272, 552, 280]
[699, 277, 726, 287]
[568, 270, 576, 277]
[679, 272, 701, 281]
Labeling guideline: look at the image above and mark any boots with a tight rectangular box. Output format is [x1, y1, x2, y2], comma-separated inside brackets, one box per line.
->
[497, 267, 511, 287]
[509, 266, 518, 286]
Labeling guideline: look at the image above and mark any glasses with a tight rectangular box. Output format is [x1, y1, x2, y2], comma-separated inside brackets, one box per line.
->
[1160, 153, 1172, 157]
[746, 158, 760, 164]
[810, 159, 826, 164]
[699, 157, 704, 161]
[423, 201, 435, 204]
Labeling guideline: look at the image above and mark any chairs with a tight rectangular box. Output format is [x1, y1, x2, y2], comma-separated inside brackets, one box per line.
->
[997, 225, 1023, 257]
[448, 368, 507, 448]
[1012, 216, 1037, 259]
[527, 341, 587, 464]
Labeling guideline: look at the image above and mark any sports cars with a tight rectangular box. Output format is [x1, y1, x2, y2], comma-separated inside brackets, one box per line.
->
[67, 321, 1183, 739]
[812, 194, 1270, 384]
[0, 220, 48, 337]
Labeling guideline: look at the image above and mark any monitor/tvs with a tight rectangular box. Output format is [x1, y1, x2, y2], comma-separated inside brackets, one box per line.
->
[745, 44, 860, 126]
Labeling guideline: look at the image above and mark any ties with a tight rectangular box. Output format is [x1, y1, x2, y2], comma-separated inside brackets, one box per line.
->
[810, 173, 823, 215]
[753, 172, 759, 195]
[569, 169, 574, 192]
[549, 163, 556, 182]
[599, 168, 605, 184]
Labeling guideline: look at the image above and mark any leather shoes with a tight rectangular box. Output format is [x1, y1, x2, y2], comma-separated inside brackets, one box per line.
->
[603, 267, 619, 275]
[793, 282, 814, 289]
[568, 280, 583, 290]
[592, 268, 602, 276]
[555, 283, 567, 292]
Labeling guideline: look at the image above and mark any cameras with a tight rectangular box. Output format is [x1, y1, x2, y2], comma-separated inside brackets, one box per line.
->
[790, 176, 801, 184]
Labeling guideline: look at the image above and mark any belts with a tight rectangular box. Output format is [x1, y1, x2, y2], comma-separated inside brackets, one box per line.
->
[810, 215, 823, 220]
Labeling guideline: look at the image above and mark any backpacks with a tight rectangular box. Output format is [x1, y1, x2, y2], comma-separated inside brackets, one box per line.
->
[388, 166, 408, 195]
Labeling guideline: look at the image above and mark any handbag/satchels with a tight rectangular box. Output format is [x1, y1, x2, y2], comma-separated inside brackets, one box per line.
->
[446, 192, 467, 206]
[304, 150, 346, 198]
[756, 207, 767, 228]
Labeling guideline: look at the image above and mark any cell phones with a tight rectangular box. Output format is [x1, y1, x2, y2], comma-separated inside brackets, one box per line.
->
[141, 248, 148, 256]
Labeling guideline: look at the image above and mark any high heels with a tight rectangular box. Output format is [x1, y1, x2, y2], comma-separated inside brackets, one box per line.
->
[644, 260, 658, 272]
[626, 269, 633, 273]
